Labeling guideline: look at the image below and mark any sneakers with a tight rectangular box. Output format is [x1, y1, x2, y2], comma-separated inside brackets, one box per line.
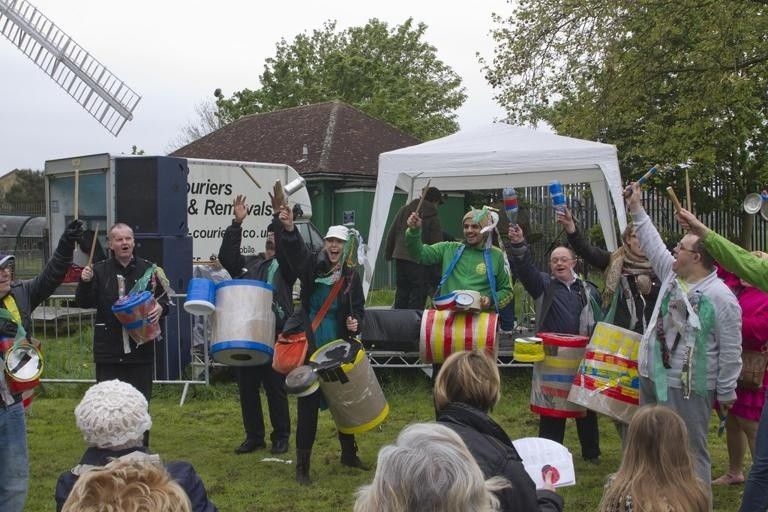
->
[270, 439, 288, 454]
[235, 438, 266, 455]
[583, 456, 600, 465]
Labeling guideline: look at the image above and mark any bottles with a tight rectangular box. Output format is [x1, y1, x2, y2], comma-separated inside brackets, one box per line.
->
[548, 181, 565, 213]
[502, 188, 519, 226]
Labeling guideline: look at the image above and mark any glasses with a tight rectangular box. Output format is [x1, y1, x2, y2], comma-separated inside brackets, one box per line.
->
[676, 241, 699, 254]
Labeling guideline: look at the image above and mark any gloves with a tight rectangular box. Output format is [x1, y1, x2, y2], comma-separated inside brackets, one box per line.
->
[59, 219, 84, 257]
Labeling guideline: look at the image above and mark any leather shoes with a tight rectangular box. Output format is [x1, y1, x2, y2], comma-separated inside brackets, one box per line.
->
[711, 472, 745, 486]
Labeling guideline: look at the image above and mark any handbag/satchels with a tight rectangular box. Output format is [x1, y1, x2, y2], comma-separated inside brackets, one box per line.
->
[738, 348, 768, 391]
[271, 332, 309, 374]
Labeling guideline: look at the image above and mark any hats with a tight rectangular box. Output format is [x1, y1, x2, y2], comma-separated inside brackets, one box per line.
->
[462, 209, 492, 239]
[423, 186, 446, 205]
[323, 225, 350, 241]
[73, 377, 153, 450]
[0, 252, 16, 268]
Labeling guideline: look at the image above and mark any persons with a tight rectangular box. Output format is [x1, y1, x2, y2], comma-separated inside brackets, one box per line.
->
[0, 217, 84, 512]
[220, 182, 304, 454]
[63, 461, 193, 512]
[55, 378, 219, 512]
[76, 223, 171, 450]
[352, 179, 768, 512]
[275, 200, 369, 487]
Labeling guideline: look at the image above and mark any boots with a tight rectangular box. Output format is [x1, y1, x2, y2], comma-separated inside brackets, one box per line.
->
[338, 438, 372, 470]
[296, 448, 313, 487]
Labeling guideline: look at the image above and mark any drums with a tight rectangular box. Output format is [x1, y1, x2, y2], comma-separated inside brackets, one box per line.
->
[183, 279, 274, 367]
[112, 290, 161, 346]
[512, 321, 643, 424]
[285, 337, 389, 434]
[5, 342, 45, 393]
[418, 291, 500, 365]
